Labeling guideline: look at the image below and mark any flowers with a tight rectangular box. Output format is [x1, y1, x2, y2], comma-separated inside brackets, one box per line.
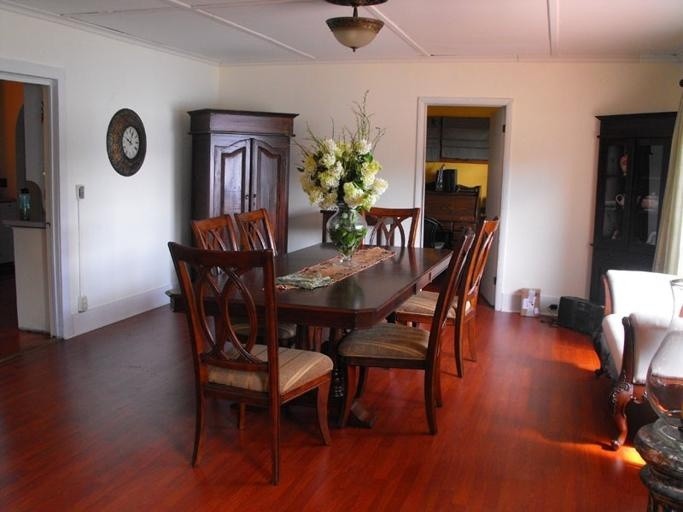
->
[284, 88, 390, 256]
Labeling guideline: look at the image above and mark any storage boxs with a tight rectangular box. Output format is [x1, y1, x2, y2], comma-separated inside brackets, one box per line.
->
[519, 288, 540, 318]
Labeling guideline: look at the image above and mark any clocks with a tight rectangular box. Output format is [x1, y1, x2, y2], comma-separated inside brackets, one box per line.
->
[105, 108, 146, 177]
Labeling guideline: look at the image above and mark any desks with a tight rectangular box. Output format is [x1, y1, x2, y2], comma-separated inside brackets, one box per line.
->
[168, 242, 454, 426]
[2, 218, 50, 335]
[425, 187, 481, 249]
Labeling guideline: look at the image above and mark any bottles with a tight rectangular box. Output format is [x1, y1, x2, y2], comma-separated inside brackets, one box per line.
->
[18, 188, 32, 221]
[435, 168, 445, 192]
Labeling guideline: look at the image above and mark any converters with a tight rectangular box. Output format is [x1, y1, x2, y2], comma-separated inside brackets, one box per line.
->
[549, 305, 558, 310]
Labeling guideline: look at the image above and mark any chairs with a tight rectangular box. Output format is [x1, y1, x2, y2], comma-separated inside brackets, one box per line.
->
[337, 229, 479, 435]
[385, 218, 502, 378]
[337, 206, 422, 355]
[234, 206, 326, 355]
[592, 269, 683, 451]
[167, 241, 335, 485]
[191, 215, 315, 390]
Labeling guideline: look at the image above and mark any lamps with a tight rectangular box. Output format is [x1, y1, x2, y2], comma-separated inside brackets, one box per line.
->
[325, 0, 389, 52]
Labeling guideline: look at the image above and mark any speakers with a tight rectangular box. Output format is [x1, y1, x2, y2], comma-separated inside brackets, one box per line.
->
[558, 295, 604, 335]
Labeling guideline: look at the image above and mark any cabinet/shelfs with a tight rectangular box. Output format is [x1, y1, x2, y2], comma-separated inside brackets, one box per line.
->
[588, 112, 677, 307]
[186, 108, 300, 255]
